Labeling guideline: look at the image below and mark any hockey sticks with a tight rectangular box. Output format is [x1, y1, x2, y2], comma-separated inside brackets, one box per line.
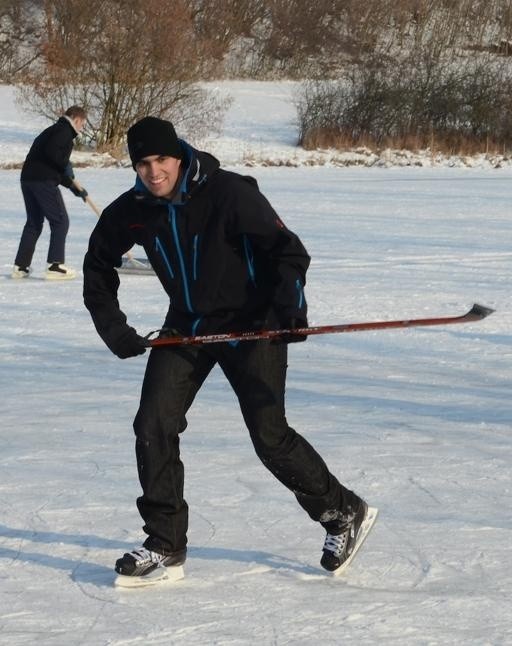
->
[147, 305, 496, 349]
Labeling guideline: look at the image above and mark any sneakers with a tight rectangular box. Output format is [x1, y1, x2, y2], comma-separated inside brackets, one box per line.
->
[318, 497, 369, 572]
[113, 543, 188, 576]
[46, 260, 74, 277]
[11, 265, 35, 274]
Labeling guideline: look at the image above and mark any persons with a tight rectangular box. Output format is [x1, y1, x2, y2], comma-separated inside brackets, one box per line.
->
[82, 117, 369, 578]
[11, 105, 89, 281]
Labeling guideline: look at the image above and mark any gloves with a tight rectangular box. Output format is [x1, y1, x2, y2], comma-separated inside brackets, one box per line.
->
[271, 316, 309, 347]
[111, 325, 149, 360]
[69, 182, 88, 203]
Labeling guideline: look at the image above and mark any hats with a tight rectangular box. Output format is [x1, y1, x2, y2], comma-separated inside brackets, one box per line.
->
[127, 114, 182, 169]
